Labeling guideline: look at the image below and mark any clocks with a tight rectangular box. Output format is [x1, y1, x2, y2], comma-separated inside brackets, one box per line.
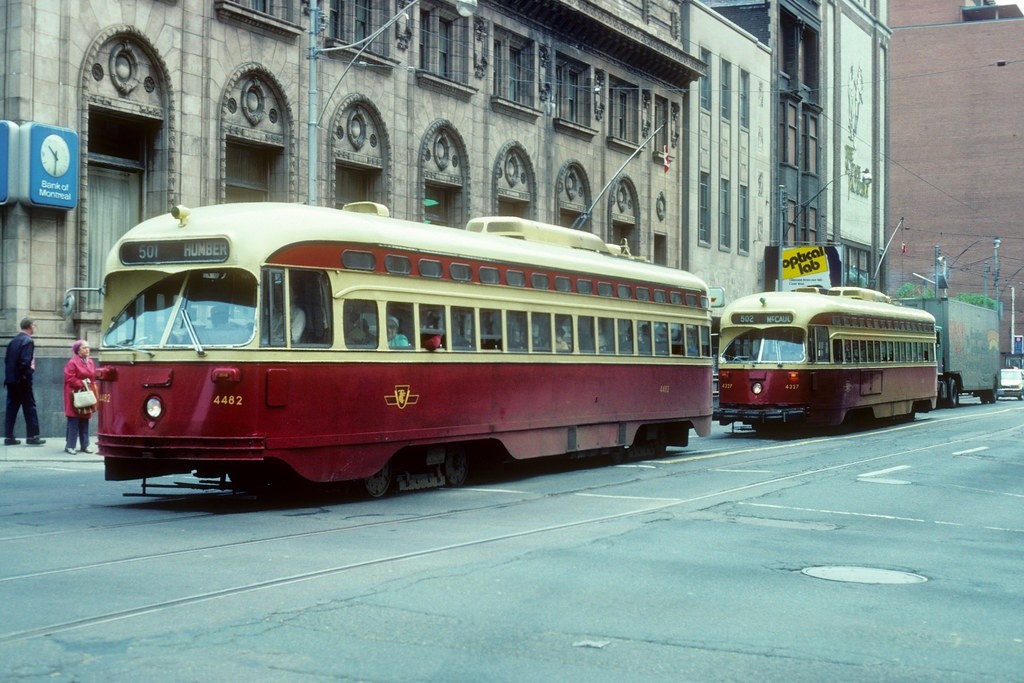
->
[41, 135, 70, 177]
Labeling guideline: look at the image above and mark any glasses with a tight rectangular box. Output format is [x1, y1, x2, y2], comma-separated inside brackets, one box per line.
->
[387, 326, 396, 329]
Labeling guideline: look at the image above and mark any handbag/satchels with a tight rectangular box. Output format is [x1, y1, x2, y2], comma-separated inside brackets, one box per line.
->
[73, 380, 97, 408]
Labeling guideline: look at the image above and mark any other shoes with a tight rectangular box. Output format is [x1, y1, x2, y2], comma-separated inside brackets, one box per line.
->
[81, 446, 93, 454]
[5, 438, 21, 445]
[66, 444, 77, 454]
[27, 436, 46, 445]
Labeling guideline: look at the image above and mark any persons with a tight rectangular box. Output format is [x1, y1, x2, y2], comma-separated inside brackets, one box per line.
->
[420, 317, 441, 351]
[3, 318, 46, 445]
[387, 316, 408, 346]
[543, 328, 568, 349]
[622, 327, 643, 351]
[322, 303, 369, 344]
[262, 284, 306, 345]
[516, 329, 528, 348]
[63, 340, 97, 454]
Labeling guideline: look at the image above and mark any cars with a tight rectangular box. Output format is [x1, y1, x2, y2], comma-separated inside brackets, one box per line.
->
[996, 368, 1024, 401]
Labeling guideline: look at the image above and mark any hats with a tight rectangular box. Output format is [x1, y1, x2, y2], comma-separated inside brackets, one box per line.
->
[388, 316, 399, 327]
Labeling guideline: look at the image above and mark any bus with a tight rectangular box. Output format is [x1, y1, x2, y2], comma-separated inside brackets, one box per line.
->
[53, 196, 719, 503]
[713, 216, 940, 439]
